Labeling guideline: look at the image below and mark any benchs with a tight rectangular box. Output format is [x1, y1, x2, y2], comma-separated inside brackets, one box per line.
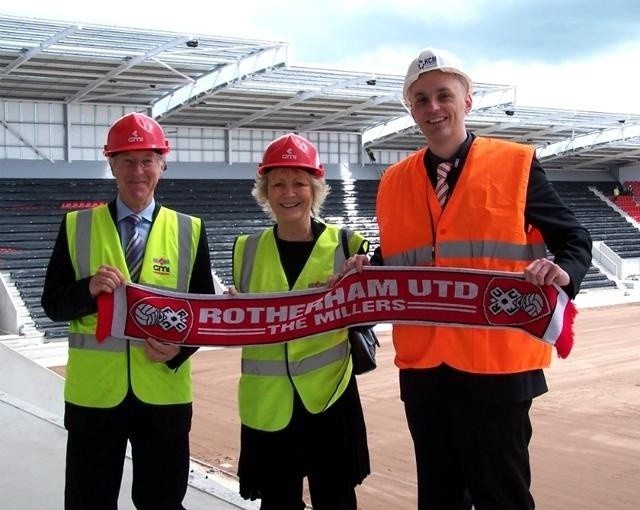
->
[0, 173, 640, 339]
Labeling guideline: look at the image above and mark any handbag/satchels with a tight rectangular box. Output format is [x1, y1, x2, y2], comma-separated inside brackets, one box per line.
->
[340, 223, 382, 375]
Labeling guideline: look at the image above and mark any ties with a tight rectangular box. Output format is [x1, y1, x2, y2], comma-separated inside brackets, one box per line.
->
[434, 160, 454, 214]
[123, 213, 146, 285]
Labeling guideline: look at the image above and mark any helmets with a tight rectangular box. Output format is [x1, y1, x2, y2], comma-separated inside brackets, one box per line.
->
[401, 48, 473, 112]
[102, 111, 170, 156]
[257, 132, 325, 180]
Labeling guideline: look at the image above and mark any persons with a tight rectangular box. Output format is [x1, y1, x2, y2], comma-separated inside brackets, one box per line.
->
[340, 49, 597, 509]
[41, 109, 219, 510]
[222, 130, 381, 510]
